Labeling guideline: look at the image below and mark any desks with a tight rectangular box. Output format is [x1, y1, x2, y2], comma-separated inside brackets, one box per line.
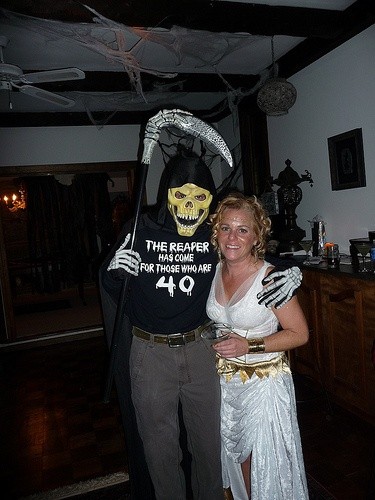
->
[9, 262, 88, 307]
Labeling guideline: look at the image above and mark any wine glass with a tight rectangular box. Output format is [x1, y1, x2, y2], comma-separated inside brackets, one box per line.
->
[299, 240, 316, 266]
[200, 323, 237, 374]
[349, 238, 373, 272]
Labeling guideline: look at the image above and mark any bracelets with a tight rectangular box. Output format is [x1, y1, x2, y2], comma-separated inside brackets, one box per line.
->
[247, 338, 265, 355]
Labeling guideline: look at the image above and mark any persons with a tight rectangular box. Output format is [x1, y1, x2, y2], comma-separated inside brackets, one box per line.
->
[206, 193, 310, 500]
[99, 144, 305, 500]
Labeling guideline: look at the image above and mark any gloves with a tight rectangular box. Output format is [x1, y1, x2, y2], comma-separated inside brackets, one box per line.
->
[256, 261, 304, 310]
[107, 231, 142, 280]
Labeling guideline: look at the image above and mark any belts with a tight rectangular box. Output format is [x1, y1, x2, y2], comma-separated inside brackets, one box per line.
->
[131, 322, 216, 348]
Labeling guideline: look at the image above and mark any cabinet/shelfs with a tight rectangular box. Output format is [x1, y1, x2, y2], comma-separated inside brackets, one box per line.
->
[269, 253, 375, 428]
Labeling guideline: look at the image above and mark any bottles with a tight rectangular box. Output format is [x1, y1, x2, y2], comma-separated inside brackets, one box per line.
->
[312, 221, 326, 256]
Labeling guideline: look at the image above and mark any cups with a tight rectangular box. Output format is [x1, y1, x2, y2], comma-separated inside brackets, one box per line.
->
[326, 242, 342, 268]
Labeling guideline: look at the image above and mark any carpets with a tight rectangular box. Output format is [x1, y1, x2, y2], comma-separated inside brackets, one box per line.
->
[23, 467, 337, 500]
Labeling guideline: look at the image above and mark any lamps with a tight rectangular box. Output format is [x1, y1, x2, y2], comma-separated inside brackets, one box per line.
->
[258, 35, 298, 116]
[3, 187, 26, 212]
[266, 159, 313, 252]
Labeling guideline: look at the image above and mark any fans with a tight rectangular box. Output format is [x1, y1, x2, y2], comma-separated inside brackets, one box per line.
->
[0, 35, 85, 108]
